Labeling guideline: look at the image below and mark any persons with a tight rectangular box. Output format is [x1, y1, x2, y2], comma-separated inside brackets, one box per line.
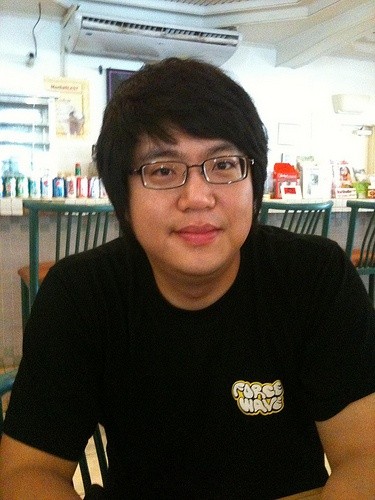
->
[0, 57, 375, 499]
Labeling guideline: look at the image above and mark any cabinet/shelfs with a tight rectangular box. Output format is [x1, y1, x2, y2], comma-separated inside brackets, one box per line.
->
[0, 93, 52, 168]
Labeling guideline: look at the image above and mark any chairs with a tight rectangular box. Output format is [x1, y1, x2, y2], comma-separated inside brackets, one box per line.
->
[257, 200, 334, 238]
[17, 199, 116, 330]
[342, 200, 375, 306]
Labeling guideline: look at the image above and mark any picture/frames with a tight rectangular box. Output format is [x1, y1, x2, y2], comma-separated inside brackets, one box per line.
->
[42, 75, 89, 142]
[107, 68, 137, 102]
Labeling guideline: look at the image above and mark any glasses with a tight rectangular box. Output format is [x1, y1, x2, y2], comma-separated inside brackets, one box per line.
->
[128, 156, 255, 190]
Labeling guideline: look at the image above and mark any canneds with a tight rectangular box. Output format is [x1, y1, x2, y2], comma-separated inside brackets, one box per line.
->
[0, 176, 109, 199]
